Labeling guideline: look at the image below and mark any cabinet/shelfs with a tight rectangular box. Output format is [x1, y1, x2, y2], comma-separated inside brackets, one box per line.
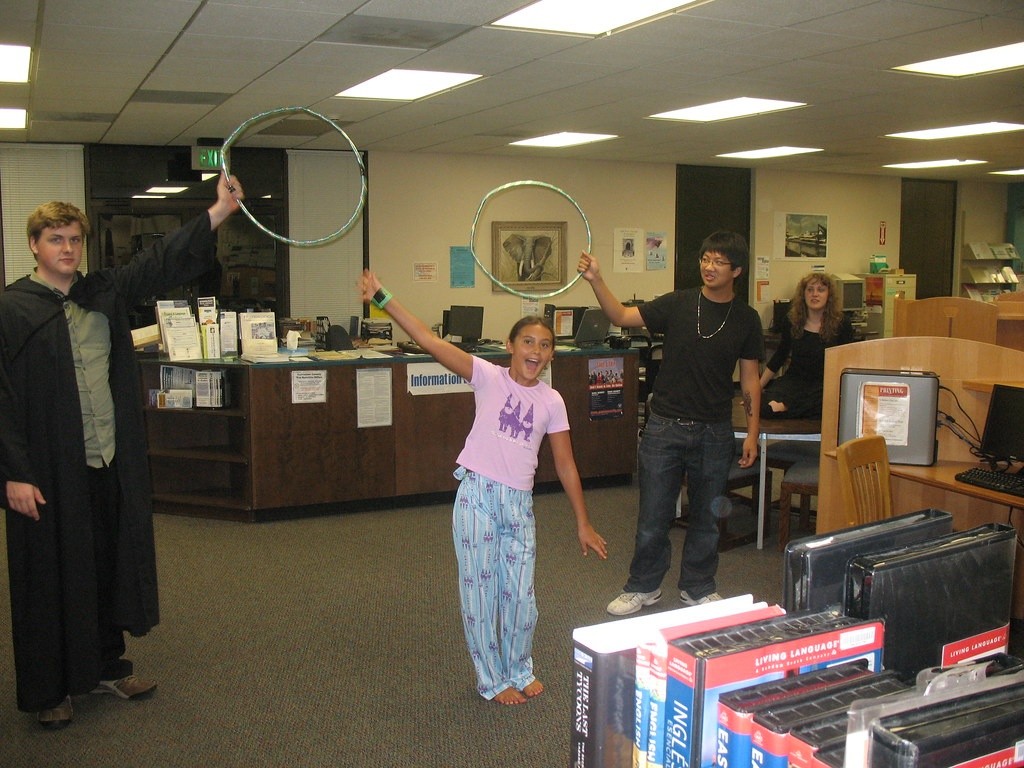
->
[136, 257, 1024, 533]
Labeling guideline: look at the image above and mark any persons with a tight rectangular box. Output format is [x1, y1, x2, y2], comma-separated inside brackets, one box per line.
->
[0, 171, 245, 722]
[577, 231, 762, 615]
[740, 273, 854, 418]
[354, 269, 607, 704]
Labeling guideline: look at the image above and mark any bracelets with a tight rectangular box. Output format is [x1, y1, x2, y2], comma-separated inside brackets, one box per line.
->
[370, 285, 392, 309]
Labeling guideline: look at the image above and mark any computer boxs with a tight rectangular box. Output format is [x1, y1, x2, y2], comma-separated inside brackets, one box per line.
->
[836, 368, 939, 466]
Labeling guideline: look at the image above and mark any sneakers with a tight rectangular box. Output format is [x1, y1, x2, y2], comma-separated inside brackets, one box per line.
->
[91, 674, 158, 698]
[35, 694, 75, 729]
[606, 587, 663, 616]
[679, 589, 724, 606]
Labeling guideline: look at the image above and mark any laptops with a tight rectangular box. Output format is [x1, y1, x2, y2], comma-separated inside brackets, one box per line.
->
[557, 309, 612, 346]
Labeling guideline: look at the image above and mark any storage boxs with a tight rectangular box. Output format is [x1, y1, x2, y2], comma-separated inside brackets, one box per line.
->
[870, 254, 887, 273]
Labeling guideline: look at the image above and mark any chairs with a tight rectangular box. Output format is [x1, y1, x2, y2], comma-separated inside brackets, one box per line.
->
[625, 334, 651, 400]
[645, 344, 663, 429]
[682, 436, 894, 552]
[326, 325, 353, 350]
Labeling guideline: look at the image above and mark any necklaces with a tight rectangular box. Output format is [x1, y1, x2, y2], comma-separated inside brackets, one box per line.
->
[698, 285, 733, 338]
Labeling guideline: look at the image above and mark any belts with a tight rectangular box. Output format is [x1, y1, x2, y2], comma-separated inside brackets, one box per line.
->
[652, 408, 713, 426]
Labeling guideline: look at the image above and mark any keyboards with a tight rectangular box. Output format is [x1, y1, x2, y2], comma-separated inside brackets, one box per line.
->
[955, 466, 1024, 498]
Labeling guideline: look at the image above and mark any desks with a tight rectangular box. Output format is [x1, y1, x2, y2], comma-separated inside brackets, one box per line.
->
[676, 397, 821, 549]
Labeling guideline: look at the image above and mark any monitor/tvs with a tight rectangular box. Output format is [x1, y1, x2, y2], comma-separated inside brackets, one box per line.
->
[448, 305, 484, 343]
[831, 272, 866, 311]
[979, 384, 1024, 476]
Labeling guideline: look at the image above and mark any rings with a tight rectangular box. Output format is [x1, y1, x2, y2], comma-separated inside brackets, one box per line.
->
[229, 187, 235, 193]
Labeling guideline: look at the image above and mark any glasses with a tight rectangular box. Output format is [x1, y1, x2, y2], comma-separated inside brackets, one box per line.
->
[699, 256, 732, 269]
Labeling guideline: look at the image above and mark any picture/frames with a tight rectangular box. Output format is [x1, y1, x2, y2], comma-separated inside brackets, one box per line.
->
[491, 221, 567, 292]
